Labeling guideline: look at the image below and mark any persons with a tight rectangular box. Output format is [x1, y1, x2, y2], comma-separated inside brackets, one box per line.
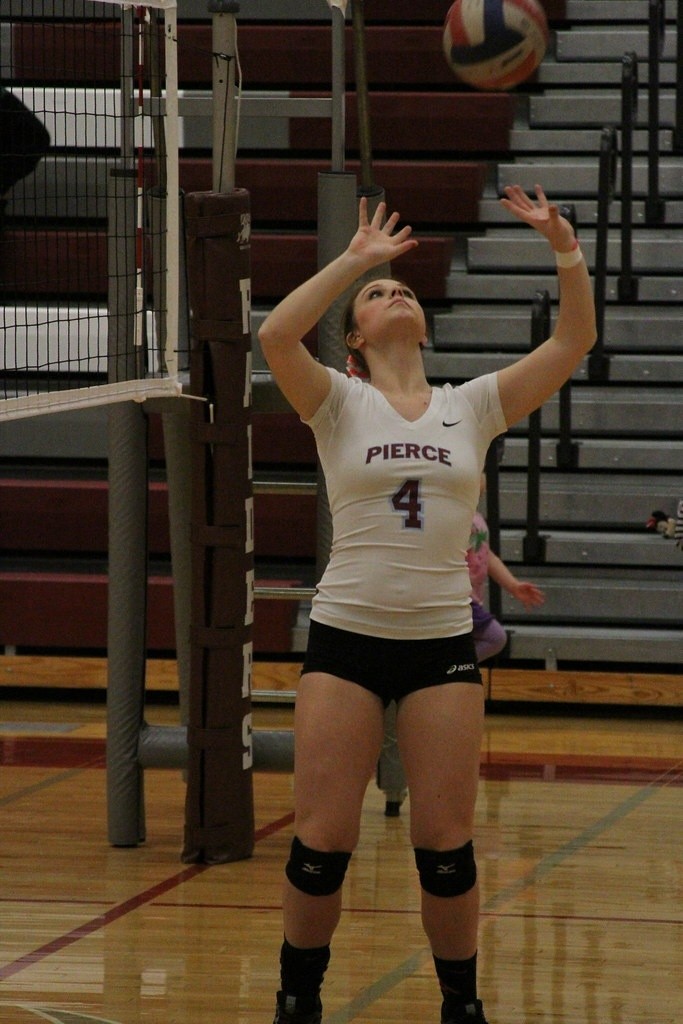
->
[258, 183, 596, 1024]
[371, 471, 545, 817]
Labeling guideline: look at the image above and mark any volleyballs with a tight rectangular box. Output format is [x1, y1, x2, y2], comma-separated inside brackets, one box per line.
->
[436, 0, 551, 92]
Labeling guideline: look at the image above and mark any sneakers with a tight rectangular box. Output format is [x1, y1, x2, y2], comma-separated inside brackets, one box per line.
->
[440, 999, 486, 1024]
[273, 991, 322, 1024]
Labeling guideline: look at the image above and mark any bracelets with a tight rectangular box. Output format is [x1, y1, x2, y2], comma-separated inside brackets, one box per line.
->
[556, 241, 582, 269]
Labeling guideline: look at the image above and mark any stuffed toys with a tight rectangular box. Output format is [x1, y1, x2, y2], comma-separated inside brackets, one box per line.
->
[647, 500, 683, 550]
